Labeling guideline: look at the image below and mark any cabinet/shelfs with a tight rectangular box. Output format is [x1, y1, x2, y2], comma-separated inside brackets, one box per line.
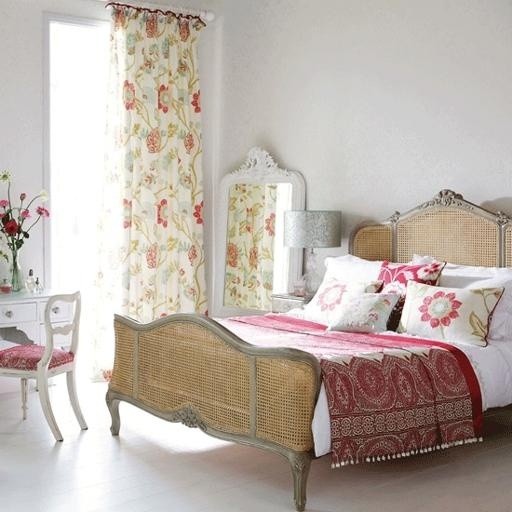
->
[0, 293, 74, 392]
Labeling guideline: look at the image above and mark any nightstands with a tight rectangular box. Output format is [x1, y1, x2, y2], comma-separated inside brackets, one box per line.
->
[271, 293, 305, 314]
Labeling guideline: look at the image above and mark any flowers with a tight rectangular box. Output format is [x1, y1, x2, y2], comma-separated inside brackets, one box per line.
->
[0, 169, 50, 269]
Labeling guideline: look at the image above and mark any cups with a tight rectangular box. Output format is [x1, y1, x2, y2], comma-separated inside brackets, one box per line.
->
[293, 281, 306, 297]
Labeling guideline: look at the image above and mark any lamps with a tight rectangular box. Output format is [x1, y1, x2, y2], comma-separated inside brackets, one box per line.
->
[284, 210, 342, 276]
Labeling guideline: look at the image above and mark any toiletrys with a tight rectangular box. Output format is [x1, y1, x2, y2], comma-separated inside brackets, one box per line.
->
[0, 278, 14, 295]
[34, 278, 44, 294]
[25, 269, 38, 293]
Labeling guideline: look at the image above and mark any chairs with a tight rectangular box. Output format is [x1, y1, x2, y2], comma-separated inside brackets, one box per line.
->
[0, 290, 88, 442]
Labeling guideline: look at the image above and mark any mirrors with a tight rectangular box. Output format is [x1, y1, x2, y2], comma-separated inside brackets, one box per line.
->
[212, 146, 306, 318]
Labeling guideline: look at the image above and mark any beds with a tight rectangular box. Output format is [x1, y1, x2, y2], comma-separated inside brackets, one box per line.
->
[103, 189, 512, 512]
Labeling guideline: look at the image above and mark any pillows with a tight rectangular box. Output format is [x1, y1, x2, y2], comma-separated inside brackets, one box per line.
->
[10, 265, 22, 292]
[299, 253, 512, 348]
